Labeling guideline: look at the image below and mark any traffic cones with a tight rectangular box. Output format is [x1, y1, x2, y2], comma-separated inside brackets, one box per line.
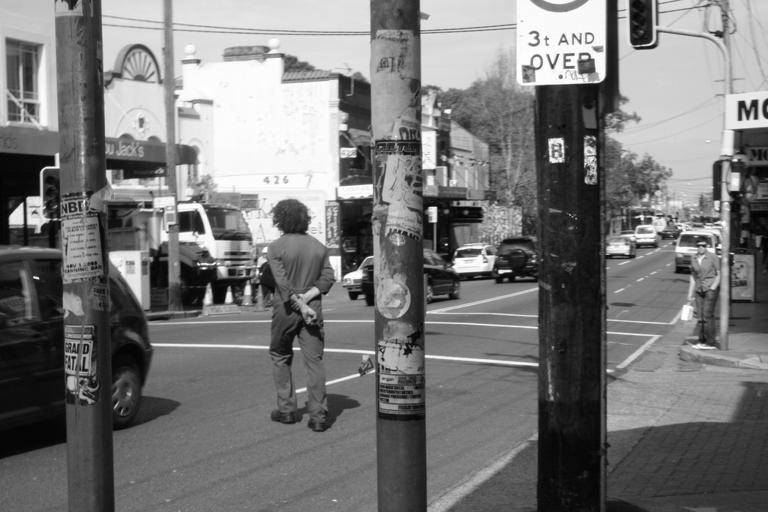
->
[240, 278, 255, 307]
[223, 284, 234, 304]
[202, 281, 214, 306]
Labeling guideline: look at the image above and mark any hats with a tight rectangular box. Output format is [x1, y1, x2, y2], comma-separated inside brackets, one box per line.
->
[261, 246, 268, 253]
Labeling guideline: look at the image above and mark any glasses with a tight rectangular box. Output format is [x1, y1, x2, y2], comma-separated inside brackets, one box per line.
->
[696, 244, 706, 247]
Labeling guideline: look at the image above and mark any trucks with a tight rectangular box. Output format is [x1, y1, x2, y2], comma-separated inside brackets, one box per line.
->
[99, 199, 258, 305]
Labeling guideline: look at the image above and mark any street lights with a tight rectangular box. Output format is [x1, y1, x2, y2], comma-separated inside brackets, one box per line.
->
[704, 137, 721, 145]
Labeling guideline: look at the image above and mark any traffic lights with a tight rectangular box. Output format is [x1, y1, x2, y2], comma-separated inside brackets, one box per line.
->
[628, 0, 657, 51]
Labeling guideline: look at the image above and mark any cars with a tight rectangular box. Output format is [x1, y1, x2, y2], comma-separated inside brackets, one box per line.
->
[343, 256, 374, 300]
[359, 245, 463, 305]
[604, 213, 722, 273]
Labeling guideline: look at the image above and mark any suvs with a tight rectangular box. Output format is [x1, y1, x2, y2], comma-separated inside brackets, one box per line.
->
[452, 241, 497, 278]
[493, 233, 539, 286]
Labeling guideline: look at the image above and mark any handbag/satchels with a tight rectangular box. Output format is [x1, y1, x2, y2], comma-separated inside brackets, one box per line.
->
[681, 304, 694, 321]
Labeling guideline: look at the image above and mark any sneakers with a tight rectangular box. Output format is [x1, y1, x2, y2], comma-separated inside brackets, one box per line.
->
[307, 420, 325, 431]
[691, 342, 716, 350]
[271, 409, 294, 423]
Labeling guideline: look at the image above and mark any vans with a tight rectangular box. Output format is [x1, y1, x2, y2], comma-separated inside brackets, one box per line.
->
[0, 243, 151, 450]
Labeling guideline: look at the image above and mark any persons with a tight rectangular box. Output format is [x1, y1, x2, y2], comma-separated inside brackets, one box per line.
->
[257, 245, 274, 306]
[686, 239, 722, 350]
[269, 199, 336, 431]
